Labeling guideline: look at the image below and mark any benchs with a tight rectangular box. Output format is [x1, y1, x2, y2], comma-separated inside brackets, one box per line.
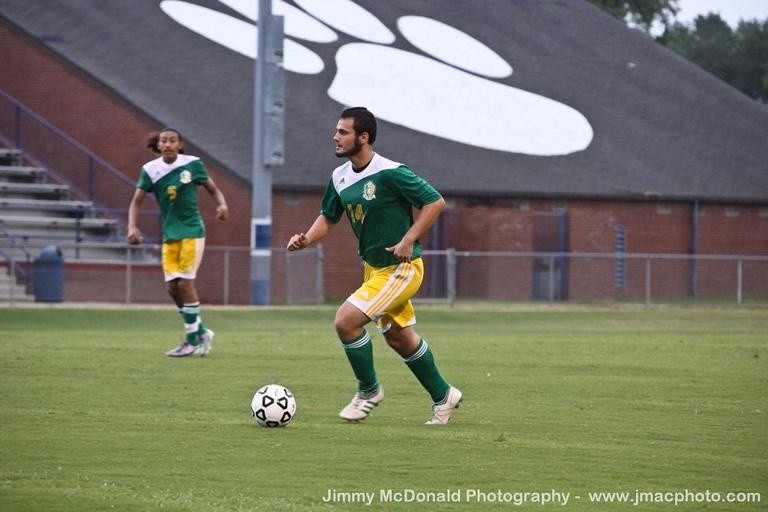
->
[0, 140, 155, 263]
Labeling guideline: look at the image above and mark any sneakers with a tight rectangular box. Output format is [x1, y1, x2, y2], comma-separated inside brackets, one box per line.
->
[338, 386, 385, 421]
[165, 327, 215, 358]
[424, 384, 462, 425]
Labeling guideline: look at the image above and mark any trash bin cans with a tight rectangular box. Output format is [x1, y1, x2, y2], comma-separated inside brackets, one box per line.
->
[34, 244, 64, 302]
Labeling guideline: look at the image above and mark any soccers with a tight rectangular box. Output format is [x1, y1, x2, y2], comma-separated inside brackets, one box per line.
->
[251, 385, 295, 427]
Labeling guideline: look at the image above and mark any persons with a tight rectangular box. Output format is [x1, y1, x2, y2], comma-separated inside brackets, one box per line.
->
[287, 107, 463, 424]
[127, 129, 228, 356]
